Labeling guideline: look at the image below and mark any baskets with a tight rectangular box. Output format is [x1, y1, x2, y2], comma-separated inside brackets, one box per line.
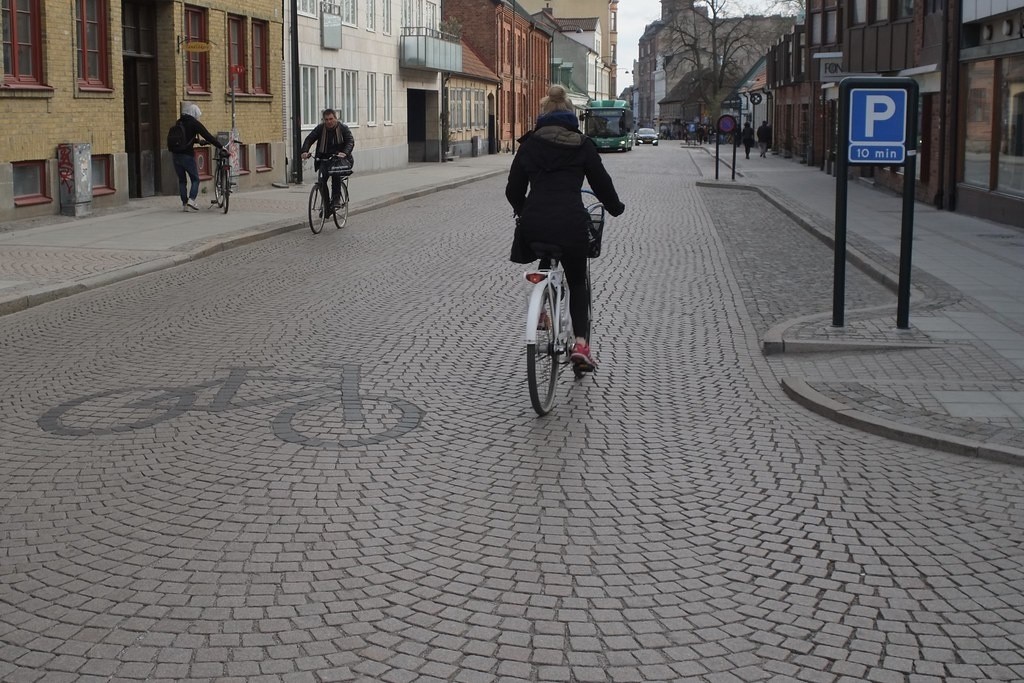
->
[583, 214, 605, 258]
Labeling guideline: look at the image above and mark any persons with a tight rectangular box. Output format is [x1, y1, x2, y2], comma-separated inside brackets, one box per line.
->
[743, 122, 754, 159]
[757, 121, 769, 158]
[173, 104, 228, 212]
[505, 84, 625, 372]
[698, 124, 708, 145]
[299, 109, 354, 218]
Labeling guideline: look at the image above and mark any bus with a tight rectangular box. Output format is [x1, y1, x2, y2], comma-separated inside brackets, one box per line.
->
[579, 101, 634, 151]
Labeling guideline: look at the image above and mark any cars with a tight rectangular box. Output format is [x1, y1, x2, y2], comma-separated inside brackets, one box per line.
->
[635, 128, 660, 146]
[686, 124, 697, 139]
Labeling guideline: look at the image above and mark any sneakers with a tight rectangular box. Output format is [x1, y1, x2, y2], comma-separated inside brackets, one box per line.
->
[569, 340, 597, 368]
[536, 312, 551, 330]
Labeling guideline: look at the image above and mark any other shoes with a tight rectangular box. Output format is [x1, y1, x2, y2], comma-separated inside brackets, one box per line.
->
[184, 206, 190, 211]
[187, 198, 200, 210]
[333, 198, 341, 207]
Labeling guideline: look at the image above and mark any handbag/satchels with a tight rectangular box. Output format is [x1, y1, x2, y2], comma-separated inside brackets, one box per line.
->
[328, 157, 353, 176]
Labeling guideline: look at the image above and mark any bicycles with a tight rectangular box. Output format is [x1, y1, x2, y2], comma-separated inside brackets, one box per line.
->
[200, 139, 243, 213]
[305, 152, 350, 234]
[524, 202, 604, 415]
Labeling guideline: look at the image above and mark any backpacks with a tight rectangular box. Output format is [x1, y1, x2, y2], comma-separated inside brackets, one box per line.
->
[166, 121, 195, 153]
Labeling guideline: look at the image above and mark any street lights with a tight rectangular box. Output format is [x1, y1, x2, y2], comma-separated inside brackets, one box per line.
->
[551, 24, 583, 84]
